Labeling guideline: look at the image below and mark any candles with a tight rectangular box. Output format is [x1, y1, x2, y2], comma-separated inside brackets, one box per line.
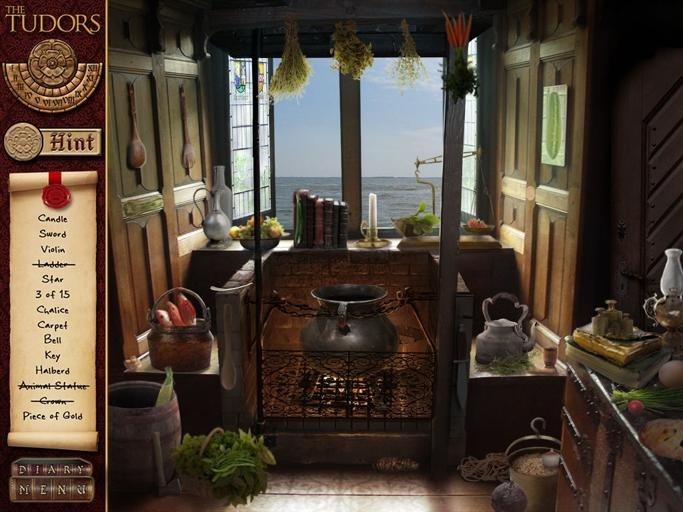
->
[368, 193, 377, 228]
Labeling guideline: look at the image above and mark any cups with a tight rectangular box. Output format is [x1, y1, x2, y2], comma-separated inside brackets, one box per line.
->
[542, 346, 557, 368]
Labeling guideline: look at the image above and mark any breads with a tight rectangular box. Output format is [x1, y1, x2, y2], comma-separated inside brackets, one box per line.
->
[639, 418, 683, 460]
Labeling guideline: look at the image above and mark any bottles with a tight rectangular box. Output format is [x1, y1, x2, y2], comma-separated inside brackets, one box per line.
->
[591, 300, 634, 338]
[214, 165, 234, 227]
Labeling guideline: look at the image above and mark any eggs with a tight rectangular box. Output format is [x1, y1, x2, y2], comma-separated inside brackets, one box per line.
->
[659, 360, 683, 387]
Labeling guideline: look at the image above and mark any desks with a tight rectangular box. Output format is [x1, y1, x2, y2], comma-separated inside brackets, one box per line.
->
[192, 237, 503, 308]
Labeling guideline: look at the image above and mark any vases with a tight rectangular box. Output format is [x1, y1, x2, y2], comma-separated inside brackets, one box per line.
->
[302, 282, 399, 379]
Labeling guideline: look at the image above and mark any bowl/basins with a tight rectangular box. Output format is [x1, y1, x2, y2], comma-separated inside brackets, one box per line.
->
[234, 233, 292, 251]
[463, 224, 496, 236]
[394, 219, 430, 237]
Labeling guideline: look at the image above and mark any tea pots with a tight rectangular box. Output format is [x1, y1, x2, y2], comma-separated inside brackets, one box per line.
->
[193, 187, 230, 242]
[475, 294, 540, 366]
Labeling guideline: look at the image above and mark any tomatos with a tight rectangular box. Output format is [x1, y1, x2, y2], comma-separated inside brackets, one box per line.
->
[628, 400, 643, 417]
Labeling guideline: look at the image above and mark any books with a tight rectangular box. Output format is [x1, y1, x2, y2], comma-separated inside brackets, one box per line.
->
[293, 190, 348, 249]
[561, 310, 671, 391]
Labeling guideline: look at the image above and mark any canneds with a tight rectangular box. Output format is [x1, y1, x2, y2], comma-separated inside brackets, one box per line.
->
[544, 347, 557, 367]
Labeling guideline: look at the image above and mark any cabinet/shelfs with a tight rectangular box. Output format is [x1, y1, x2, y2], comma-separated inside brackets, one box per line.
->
[107, 59, 218, 370]
[555, 363, 683, 512]
[492, 25, 608, 354]
